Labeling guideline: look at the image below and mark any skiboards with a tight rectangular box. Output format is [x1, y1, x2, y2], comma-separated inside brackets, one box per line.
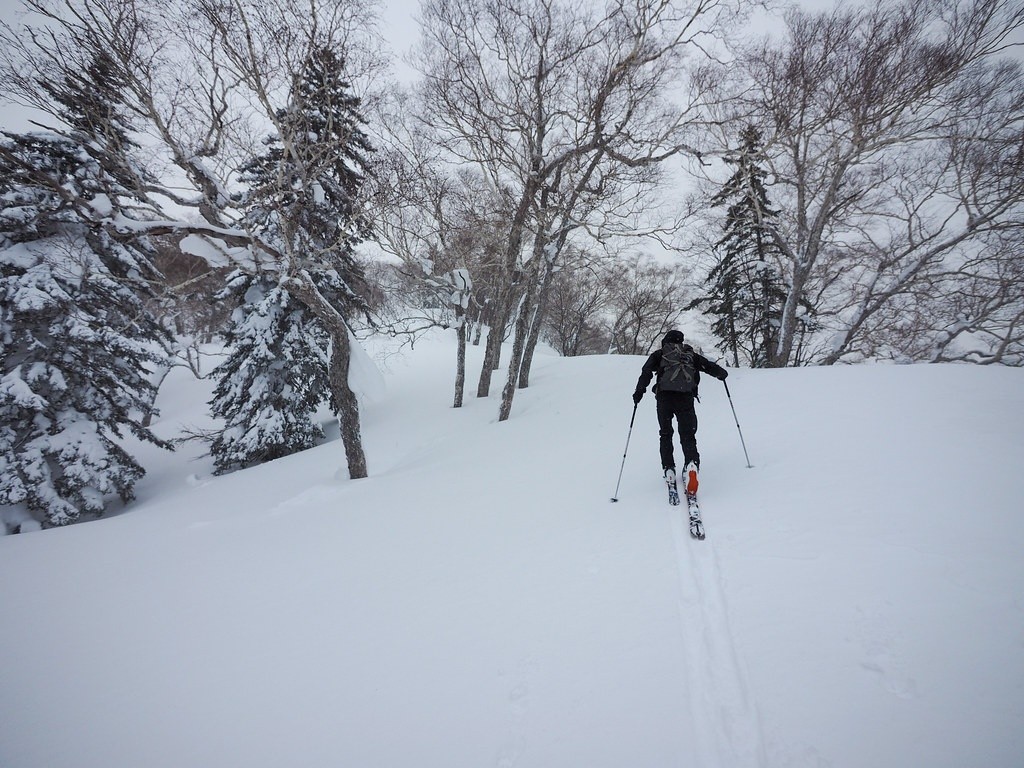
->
[666, 463, 706, 539]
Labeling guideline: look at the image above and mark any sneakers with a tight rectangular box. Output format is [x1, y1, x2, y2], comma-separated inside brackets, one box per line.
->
[685, 462, 699, 495]
[664, 468, 676, 483]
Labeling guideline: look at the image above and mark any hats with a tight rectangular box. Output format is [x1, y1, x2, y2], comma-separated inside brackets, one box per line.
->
[663, 330, 684, 343]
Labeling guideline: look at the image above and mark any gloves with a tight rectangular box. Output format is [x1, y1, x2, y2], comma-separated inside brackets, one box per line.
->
[717, 371, 728, 380]
[632, 391, 643, 404]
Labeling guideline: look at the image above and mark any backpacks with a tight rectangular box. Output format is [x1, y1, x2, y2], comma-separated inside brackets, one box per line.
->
[658, 343, 695, 392]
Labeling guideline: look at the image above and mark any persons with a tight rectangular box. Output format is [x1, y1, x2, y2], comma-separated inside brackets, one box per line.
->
[633, 329, 728, 495]
